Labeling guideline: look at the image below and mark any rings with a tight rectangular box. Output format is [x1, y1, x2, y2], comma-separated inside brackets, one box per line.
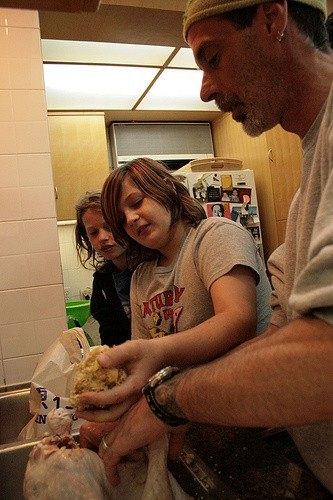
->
[101, 437, 109, 451]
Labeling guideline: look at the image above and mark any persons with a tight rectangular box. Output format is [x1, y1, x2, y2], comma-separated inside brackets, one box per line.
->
[211, 204, 224, 217]
[221, 189, 241, 202]
[74, 158, 294, 500]
[80, 0, 333, 494]
[75, 192, 145, 346]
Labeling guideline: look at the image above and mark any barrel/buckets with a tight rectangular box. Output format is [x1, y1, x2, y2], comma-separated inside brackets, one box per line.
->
[65, 300, 94, 348]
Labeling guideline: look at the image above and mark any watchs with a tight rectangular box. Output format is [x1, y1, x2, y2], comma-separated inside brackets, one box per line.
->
[141, 366, 189, 426]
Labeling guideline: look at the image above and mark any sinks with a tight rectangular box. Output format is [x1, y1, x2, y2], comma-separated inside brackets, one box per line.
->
[0, 381, 82, 455]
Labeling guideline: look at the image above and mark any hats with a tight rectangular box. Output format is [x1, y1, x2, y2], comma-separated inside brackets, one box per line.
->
[182, 1, 327, 43]
